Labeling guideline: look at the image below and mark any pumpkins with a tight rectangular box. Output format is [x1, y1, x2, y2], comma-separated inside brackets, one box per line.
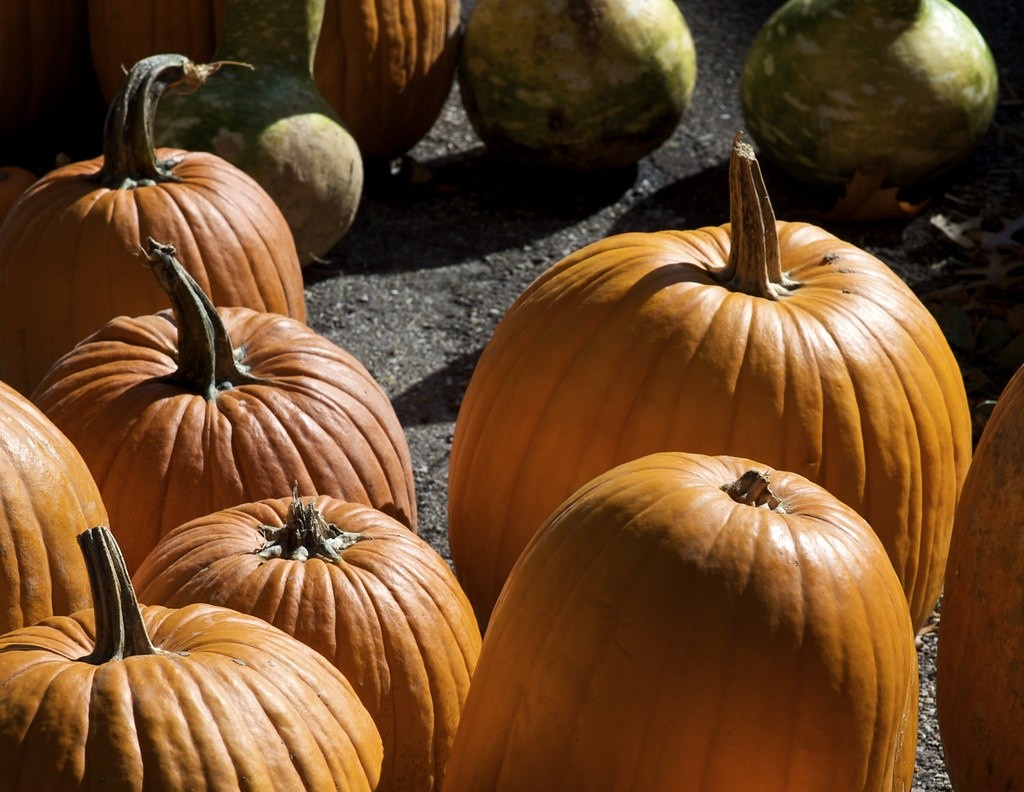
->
[0, 0, 1024, 792]
